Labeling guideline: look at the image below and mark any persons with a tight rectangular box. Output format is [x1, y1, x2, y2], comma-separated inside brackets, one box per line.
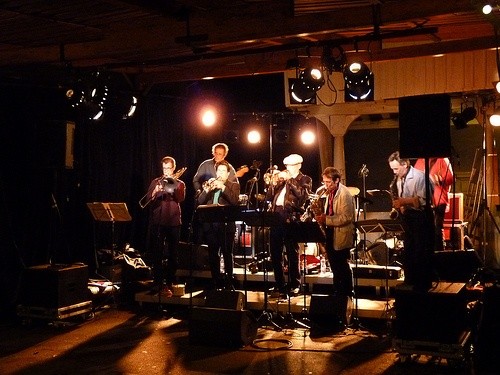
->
[266, 154, 312, 298]
[193, 143, 239, 193]
[197, 160, 240, 292]
[313, 167, 355, 297]
[413, 157, 453, 251]
[388, 151, 433, 291]
[146, 156, 186, 295]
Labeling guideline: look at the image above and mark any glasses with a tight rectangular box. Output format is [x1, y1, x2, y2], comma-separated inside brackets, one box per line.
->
[321, 181, 335, 184]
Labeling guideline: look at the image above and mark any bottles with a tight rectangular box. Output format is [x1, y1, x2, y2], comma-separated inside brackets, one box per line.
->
[320, 256, 327, 275]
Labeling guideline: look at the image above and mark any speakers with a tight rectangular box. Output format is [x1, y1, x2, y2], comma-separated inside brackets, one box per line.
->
[398, 94, 451, 158]
[310, 293, 346, 336]
[189, 306, 257, 348]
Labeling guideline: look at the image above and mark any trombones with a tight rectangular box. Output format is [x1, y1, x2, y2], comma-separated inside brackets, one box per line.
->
[138, 167, 188, 209]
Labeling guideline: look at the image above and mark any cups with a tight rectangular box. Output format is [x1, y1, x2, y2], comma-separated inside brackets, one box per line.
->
[49, 255, 57, 265]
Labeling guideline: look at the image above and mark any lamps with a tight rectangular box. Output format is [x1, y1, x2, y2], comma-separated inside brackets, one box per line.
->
[289, 65, 326, 105]
[343, 61, 375, 102]
[451, 96, 477, 129]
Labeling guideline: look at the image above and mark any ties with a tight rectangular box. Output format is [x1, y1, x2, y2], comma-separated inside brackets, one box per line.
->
[329, 188, 338, 228]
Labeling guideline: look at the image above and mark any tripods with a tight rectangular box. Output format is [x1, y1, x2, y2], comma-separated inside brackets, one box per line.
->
[469, 110, 500, 265]
[242, 208, 326, 331]
[345, 224, 371, 332]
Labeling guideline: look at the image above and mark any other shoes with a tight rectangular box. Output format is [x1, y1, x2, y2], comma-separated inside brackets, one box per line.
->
[290, 287, 300, 294]
[268, 286, 279, 290]
[151, 282, 160, 292]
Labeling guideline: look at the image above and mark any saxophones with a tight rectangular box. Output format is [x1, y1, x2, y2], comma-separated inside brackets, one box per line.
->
[300, 188, 331, 237]
[388, 175, 405, 221]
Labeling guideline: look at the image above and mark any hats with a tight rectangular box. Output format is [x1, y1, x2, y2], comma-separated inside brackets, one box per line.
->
[283, 154, 303, 164]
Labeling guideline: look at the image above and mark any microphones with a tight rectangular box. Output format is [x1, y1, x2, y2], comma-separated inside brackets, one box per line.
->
[268, 165, 278, 170]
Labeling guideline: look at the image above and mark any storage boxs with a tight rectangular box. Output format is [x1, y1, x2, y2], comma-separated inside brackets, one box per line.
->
[444, 193, 464, 223]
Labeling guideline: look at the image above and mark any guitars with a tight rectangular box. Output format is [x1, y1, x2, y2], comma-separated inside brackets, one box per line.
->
[232, 164, 249, 181]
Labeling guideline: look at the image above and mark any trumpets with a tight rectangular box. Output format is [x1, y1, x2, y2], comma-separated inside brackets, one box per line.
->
[201, 175, 222, 194]
[263, 169, 292, 190]
